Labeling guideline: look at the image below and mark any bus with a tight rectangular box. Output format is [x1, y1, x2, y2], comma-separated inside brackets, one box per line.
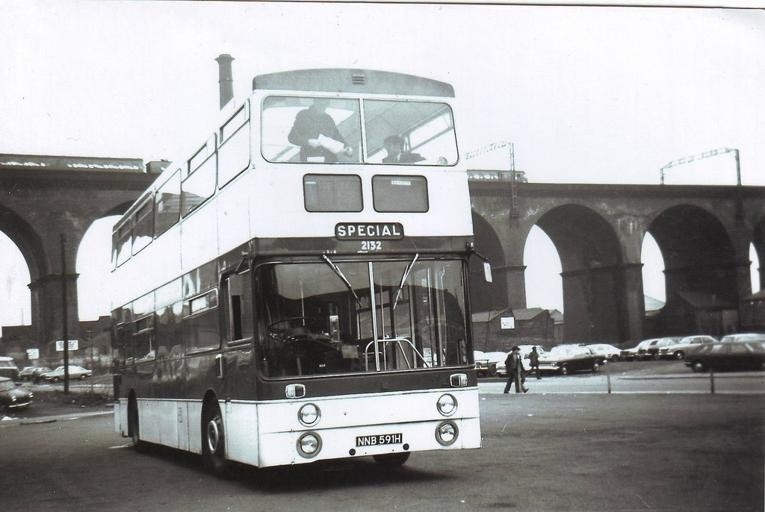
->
[107, 67, 486, 481]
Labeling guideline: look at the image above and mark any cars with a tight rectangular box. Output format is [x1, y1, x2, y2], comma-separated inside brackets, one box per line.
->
[114, 336, 259, 393]
[0, 357, 92, 413]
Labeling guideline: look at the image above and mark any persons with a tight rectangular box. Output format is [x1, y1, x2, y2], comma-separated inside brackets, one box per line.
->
[524, 346, 542, 380]
[381, 135, 426, 164]
[286, 97, 354, 164]
[503, 345, 529, 394]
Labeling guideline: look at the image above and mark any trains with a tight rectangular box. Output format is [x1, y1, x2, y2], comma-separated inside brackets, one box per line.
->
[1, 151, 531, 186]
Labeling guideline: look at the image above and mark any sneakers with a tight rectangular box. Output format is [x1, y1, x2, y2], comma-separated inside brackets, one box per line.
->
[503, 387, 530, 394]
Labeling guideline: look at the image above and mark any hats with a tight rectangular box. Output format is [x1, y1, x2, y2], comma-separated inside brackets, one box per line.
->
[511, 345, 521, 351]
